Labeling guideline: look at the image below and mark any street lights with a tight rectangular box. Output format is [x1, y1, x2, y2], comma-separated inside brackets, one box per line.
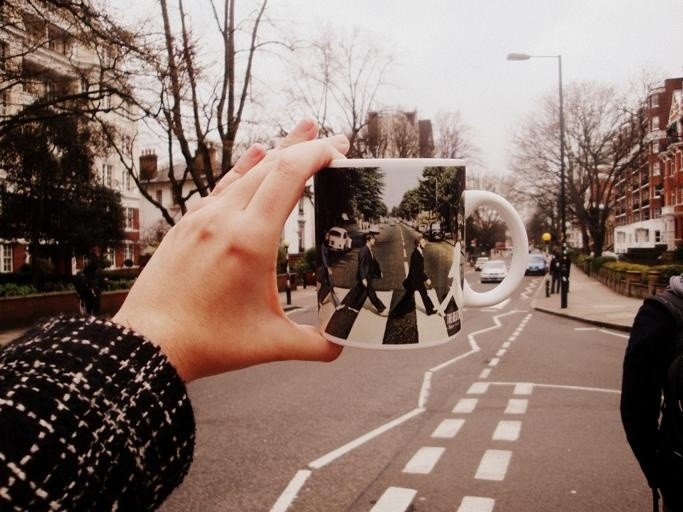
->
[541, 232, 553, 257]
[425, 175, 440, 221]
[505, 51, 568, 310]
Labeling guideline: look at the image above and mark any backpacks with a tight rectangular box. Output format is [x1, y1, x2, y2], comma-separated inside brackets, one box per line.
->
[644, 290, 683, 511]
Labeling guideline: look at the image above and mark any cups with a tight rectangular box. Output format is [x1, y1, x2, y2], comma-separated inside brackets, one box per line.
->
[309, 155, 530, 352]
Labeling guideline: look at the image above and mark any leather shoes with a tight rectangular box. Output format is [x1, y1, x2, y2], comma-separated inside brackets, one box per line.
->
[334, 304, 359, 313]
[426, 309, 447, 317]
[378, 308, 405, 320]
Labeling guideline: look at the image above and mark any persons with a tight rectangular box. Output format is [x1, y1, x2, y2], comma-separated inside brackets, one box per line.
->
[620, 272, 683, 512]
[550, 253, 570, 294]
[75, 261, 100, 316]
[318, 229, 463, 317]
[0, 116, 351, 512]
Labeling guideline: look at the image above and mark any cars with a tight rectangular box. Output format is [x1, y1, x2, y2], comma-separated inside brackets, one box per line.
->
[325, 225, 351, 253]
[474, 256, 490, 271]
[479, 260, 508, 283]
[422, 220, 445, 243]
[523, 253, 549, 276]
